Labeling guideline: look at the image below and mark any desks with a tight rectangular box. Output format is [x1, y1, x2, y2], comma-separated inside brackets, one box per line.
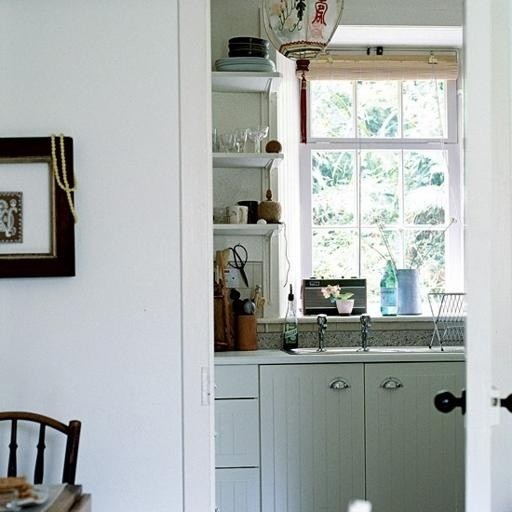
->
[1, 485, 92, 512]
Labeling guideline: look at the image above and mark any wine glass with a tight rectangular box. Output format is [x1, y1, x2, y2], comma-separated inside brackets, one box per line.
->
[220, 124, 269, 153]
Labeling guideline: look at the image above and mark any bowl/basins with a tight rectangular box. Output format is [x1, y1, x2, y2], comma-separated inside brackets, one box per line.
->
[228, 37, 269, 57]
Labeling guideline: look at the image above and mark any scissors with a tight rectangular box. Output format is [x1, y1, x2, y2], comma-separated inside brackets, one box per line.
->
[225, 245, 249, 288]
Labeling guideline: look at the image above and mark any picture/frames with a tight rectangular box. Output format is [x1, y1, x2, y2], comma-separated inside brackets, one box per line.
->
[0, 138, 74, 278]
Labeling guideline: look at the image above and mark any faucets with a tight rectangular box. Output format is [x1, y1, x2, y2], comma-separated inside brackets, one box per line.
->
[359, 313, 372, 351]
[316, 314, 327, 350]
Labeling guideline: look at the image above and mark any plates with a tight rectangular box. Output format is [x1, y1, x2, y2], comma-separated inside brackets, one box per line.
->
[215, 57, 274, 72]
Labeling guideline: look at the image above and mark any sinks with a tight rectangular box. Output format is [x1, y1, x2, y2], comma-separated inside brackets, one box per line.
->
[291, 350, 383, 354]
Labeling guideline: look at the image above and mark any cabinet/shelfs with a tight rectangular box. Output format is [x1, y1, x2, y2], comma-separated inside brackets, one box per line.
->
[261, 362, 466, 512]
[211, 70, 285, 235]
[214, 365, 261, 512]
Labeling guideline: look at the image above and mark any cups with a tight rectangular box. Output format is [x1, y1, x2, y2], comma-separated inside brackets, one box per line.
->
[213, 200, 258, 224]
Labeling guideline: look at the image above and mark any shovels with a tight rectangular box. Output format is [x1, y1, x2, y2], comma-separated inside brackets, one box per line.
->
[216, 249, 228, 286]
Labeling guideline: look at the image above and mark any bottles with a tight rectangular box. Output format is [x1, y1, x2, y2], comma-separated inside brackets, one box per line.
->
[380, 260, 397, 315]
[283, 284, 298, 350]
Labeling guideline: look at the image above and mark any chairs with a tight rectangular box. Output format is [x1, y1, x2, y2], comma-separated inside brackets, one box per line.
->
[0, 411, 81, 486]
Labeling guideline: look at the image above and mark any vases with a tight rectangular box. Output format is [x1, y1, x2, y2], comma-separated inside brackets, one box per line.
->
[335, 299, 355, 316]
[396, 267, 422, 314]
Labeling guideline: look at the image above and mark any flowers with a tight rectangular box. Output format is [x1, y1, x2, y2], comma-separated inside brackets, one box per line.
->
[363, 215, 458, 274]
[320, 284, 354, 303]
